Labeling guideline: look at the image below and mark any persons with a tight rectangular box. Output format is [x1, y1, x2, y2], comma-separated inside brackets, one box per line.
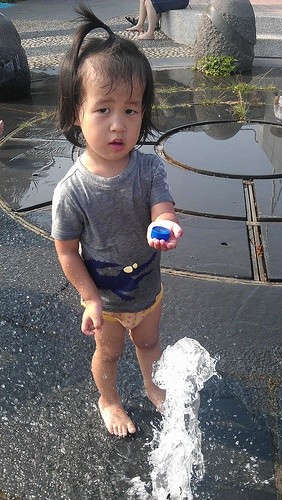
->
[125, 0, 189, 40]
[51, 3, 182, 438]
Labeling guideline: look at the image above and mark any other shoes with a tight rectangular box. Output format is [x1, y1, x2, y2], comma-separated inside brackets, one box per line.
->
[125, 16, 139, 26]
[155, 22, 160, 30]
